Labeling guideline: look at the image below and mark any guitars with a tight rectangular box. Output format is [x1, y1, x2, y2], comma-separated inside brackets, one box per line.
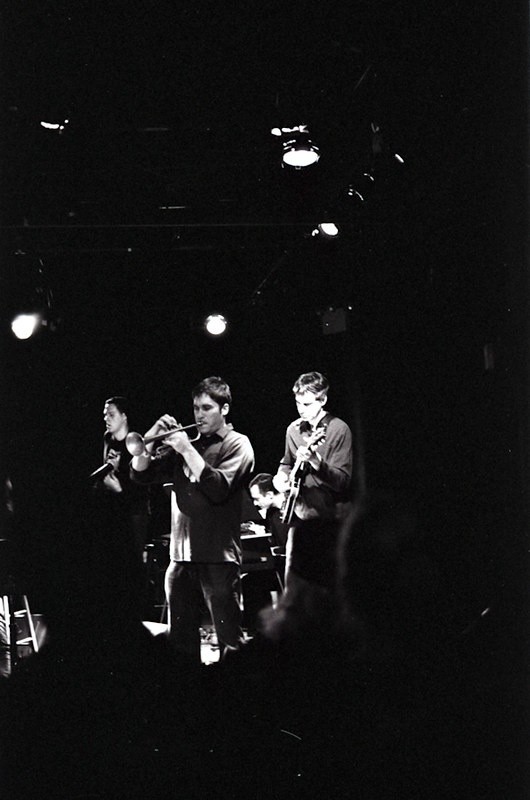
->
[280, 423, 327, 525]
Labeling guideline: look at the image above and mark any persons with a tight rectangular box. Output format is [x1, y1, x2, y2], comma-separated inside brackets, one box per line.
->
[130, 376, 255, 660]
[242, 473, 290, 640]
[103, 397, 148, 543]
[273, 372, 353, 626]
[0, 495, 494, 800]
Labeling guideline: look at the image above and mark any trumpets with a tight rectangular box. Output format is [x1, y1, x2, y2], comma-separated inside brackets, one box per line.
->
[126, 422, 204, 458]
